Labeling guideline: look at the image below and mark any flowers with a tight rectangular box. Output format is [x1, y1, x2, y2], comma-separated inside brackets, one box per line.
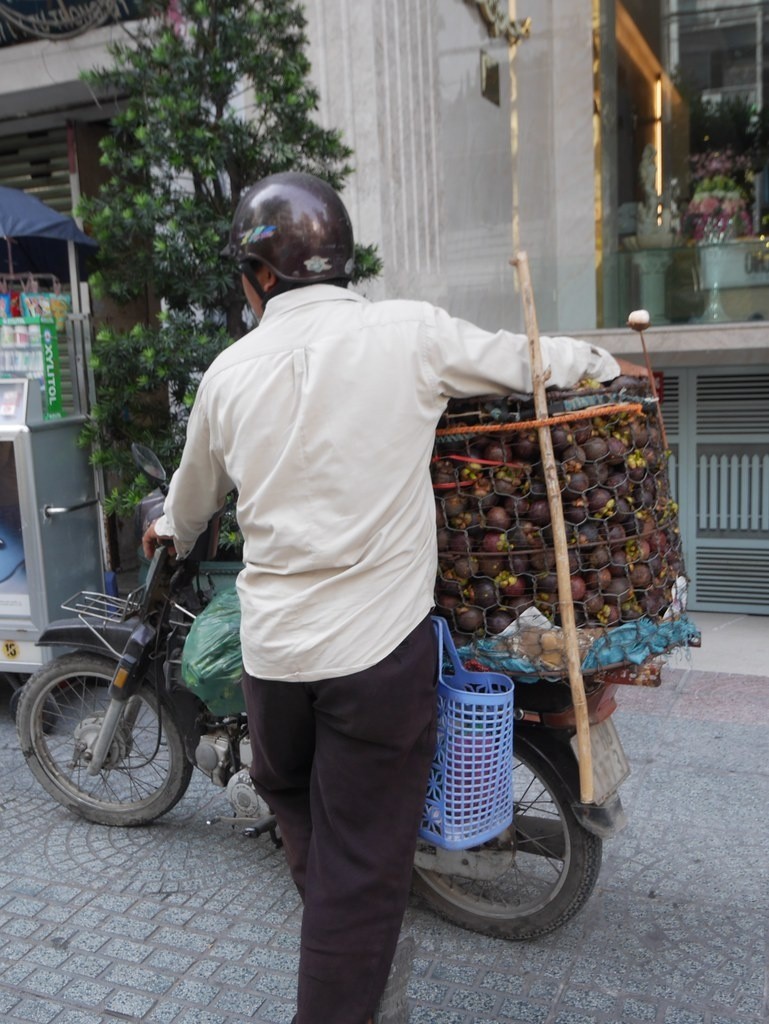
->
[689, 150, 754, 246]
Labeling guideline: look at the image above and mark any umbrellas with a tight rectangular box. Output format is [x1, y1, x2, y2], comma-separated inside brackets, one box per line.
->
[0, 186, 102, 288]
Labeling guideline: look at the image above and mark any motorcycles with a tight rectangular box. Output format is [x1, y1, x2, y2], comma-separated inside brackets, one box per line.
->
[14, 438, 702, 941]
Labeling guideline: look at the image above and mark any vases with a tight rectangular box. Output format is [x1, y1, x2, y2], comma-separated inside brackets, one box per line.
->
[692, 217, 735, 323]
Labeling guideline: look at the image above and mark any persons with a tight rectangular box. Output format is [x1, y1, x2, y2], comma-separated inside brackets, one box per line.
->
[143, 173, 652, 1024]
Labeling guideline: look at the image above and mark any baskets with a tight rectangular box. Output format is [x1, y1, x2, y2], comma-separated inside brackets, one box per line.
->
[417, 615, 514, 850]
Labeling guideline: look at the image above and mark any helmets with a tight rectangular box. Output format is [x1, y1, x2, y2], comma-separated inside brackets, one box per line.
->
[219, 171, 354, 284]
